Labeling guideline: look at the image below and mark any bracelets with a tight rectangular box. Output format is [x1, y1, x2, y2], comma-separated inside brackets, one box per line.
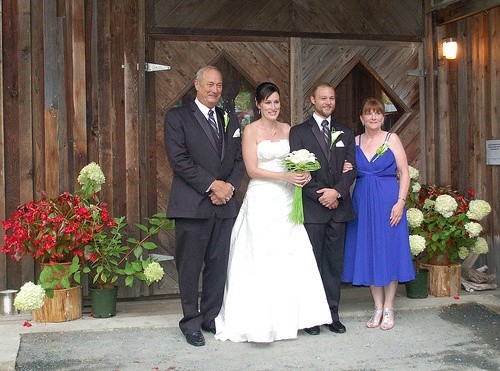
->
[399, 197, 405, 202]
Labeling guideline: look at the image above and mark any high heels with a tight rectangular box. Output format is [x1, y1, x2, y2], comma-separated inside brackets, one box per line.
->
[365, 307, 383, 329]
[380, 307, 394, 330]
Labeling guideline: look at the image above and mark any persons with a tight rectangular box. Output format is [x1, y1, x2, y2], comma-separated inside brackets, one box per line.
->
[342, 98, 415, 331]
[164, 65, 246, 347]
[214, 82, 333, 343]
[288, 83, 357, 336]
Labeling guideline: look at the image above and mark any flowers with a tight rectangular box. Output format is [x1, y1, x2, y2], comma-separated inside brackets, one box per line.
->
[376, 142, 389, 157]
[402, 165, 491, 263]
[224, 110, 229, 132]
[0, 162, 176, 310]
[284, 148, 322, 224]
[328, 127, 344, 148]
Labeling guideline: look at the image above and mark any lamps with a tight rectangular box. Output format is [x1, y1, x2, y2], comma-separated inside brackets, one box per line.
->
[443, 37, 458, 60]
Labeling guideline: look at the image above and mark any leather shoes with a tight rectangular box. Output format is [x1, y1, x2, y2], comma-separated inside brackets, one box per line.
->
[202, 325, 217, 334]
[304, 326, 320, 335]
[185, 331, 205, 346]
[324, 321, 346, 333]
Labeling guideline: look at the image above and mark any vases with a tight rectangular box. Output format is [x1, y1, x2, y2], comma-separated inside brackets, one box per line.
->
[40, 262, 72, 290]
[89, 286, 119, 318]
[429, 249, 450, 265]
[405, 270, 430, 299]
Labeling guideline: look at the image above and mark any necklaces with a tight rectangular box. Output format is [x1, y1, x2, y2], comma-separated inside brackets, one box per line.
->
[262, 119, 278, 136]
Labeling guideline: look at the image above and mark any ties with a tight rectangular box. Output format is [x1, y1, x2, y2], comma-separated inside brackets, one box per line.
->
[207, 110, 222, 158]
[321, 120, 330, 151]
